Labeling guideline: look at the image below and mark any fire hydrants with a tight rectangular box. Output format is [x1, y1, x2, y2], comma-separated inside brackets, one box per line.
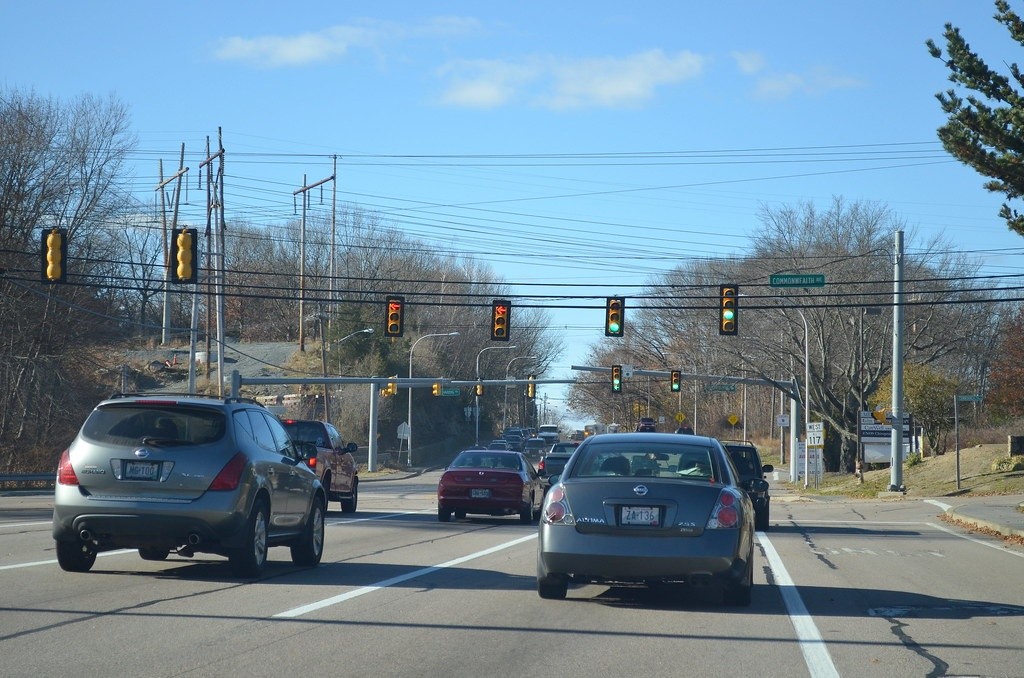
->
[855, 461, 864, 484]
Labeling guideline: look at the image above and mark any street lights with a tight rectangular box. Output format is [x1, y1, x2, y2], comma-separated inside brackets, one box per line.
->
[854, 307, 884, 460]
[739, 293, 811, 490]
[475, 346, 516, 448]
[502, 356, 540, 434]
[405, 331, 461, 468]
[662, 352, 697, 436]
[324, 328, 375, 423]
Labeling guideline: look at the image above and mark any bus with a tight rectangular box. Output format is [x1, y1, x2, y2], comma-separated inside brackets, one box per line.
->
[607, 423, 621, 433]
[585, 424, 610, 435]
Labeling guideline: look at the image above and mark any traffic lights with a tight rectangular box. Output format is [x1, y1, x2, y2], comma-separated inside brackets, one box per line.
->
[388, 374, 398, 395]
[719, 284, 740, 336]
[671, 370, 681, 392]
[491, 300, 511, 341]
[612, 365, 622, 393]
[432, 383, 442, 396]
[605, 297, 626, 337]
[384, 296, 404, 337]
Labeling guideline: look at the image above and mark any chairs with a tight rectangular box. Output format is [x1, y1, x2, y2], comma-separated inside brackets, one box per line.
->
[599, 456, 632, 477]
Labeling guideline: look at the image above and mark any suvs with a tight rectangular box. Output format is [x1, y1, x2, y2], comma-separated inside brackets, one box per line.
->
[52, 393, 328, 581]
[635, 418, 658, 432]
[536, 424, 562, 444]
[281, 419, 361, 513]
[675, 426, 694, 435]
[678, 439, 775, 530]
[488, 426, 548, 457]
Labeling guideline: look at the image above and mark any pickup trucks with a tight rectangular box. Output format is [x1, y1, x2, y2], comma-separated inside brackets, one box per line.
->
[538, 441, 585, 486]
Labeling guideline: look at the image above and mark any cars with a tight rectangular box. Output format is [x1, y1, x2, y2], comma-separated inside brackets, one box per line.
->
[569, 430, 585, 440]
[436, 448, 548, 522]
[535, 433, 769, 602]
